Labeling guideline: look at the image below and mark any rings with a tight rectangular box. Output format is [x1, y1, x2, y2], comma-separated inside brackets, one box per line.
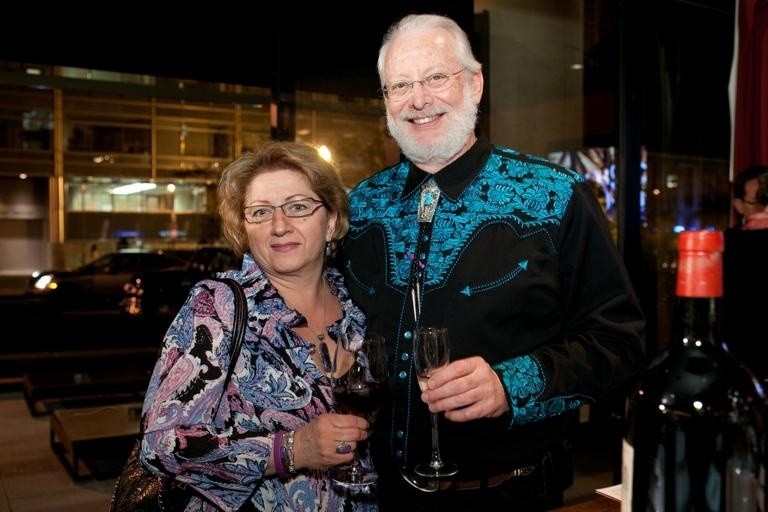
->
[336, 441, 353, 454]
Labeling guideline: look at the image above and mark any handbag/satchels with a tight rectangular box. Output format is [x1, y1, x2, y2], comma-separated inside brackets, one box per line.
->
[114, 438, 190, 512]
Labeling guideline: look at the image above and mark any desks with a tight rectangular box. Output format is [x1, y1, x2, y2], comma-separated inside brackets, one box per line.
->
[544, 489, 620, 512]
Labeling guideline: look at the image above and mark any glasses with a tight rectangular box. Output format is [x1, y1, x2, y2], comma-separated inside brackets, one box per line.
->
[239, 197, 326, 224]
[380, 68, 471, 103]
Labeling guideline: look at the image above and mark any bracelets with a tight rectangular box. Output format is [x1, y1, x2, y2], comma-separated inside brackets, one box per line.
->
[274, 429, 296, 479]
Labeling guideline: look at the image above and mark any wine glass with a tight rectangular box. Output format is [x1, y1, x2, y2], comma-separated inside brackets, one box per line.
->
[329, 334, 389, 492]
[412, 325, 459, 482]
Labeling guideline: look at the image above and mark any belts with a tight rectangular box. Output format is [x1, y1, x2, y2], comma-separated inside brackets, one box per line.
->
[422, 465, 545, 495]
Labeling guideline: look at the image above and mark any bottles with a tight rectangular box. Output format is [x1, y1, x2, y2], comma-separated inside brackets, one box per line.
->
[623, 230, 766, 512]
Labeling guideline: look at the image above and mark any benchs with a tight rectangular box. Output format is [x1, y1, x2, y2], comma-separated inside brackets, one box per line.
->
[20, 351, 158, 417]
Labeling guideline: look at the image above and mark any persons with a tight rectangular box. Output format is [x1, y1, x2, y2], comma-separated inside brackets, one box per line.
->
[323, 15, 648, 511]
[731, 164, 768, 228]
[90, 243, 99, 263]
[716, 171, 768, 381]
[117, 236, 128, 249]
[142, 141, 387, 511]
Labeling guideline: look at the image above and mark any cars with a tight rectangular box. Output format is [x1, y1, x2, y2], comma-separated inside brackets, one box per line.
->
[26, 244, 239, 344]
